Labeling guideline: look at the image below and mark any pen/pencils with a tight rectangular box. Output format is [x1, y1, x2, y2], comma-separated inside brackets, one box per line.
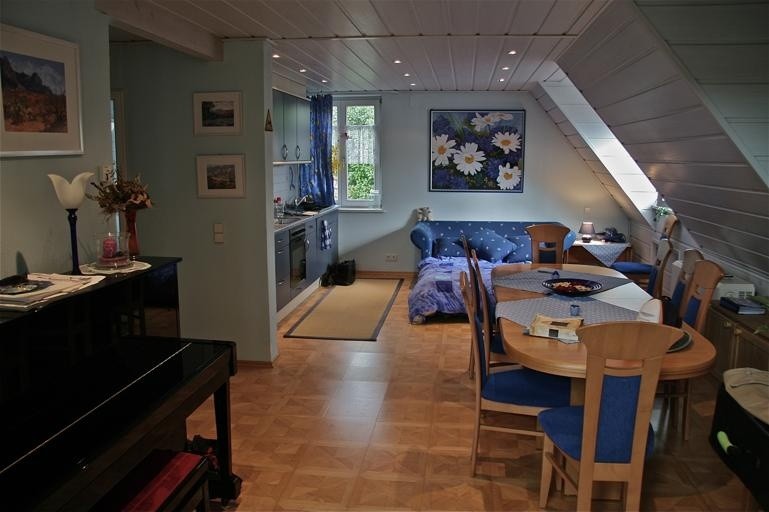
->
[538, 271, 551, 273]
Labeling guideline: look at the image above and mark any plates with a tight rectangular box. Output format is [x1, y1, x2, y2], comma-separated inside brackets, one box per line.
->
[540, 276, 604, 298]
[665, 329, 693, 354]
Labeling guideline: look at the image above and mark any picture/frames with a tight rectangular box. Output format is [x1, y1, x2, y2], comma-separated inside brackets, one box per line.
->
[0, 20, 86, 159]
[429, 107, 526, 194]
[192, 90, 243, 138]
[194, 151, 249, 201]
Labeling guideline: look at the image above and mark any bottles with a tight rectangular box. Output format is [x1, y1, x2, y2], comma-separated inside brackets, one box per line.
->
[272, 200, 277, 223]
[550, 271, 560, 280]
[277, 196, 284, 217]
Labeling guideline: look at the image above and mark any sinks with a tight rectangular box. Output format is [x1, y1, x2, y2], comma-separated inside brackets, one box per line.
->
[301, 209, 320, 216]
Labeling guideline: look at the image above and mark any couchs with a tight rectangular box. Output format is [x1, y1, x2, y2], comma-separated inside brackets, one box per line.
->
[408, 218, 578, 262]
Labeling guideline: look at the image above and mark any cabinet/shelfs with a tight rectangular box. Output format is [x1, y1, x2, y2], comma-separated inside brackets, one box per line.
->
[275, 229, 292, 324]
[296, 94, 310, 164]
[270, 87, 299, 164]
[316, 207, 340, 287]
[709, 386, 767, 508]
[705, 301, 768, 383]
[303, 218, 320, 296]
[290, 279, 310, 310]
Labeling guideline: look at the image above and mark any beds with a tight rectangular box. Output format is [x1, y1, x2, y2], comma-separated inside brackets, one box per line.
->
[406, 258, 574, 325]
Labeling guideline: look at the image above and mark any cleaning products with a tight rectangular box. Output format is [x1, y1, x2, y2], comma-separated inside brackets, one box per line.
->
[273, 197, 284, 223]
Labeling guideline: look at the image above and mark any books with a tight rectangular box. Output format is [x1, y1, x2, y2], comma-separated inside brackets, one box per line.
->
[719, 296, 766, 315]
[0, 270, 84, 311]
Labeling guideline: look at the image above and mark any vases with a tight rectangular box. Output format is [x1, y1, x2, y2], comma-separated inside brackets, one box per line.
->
[126, 218, 140, 255]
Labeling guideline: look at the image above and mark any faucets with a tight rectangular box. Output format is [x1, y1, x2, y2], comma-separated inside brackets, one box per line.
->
[297, 197, 308, 207]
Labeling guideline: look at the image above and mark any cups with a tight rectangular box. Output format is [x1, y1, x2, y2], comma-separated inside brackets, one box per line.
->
[94, 232, 132, 268]
[569, 304, 581, 317]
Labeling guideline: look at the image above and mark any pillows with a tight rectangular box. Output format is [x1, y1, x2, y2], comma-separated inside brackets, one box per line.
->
[433, 233, 468, 258]
[468, 226, 517, 262]
[506, 233, 542, 263]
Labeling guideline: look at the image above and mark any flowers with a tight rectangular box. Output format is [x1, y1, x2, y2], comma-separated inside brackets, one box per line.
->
[89, 161, 153, 216]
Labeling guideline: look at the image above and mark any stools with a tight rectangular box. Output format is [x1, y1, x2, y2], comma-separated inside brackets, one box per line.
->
[112, 447, 210, 510]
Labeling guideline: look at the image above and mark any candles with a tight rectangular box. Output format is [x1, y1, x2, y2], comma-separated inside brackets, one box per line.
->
[101, 235, 115, 259]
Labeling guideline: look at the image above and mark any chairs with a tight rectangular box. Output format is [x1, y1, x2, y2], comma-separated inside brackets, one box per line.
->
[459, 225, 725, 512]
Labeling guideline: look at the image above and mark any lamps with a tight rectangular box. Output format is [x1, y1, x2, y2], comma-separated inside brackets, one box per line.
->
[44, 166, 100, 276]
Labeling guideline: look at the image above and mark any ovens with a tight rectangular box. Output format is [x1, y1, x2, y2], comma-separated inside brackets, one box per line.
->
[288, 222, 307, 289]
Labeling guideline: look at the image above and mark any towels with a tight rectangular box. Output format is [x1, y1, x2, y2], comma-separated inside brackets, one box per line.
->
[320, 220, 333, 252]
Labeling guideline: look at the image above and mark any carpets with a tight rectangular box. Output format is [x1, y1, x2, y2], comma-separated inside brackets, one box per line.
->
[283, 276, 404, 341]
